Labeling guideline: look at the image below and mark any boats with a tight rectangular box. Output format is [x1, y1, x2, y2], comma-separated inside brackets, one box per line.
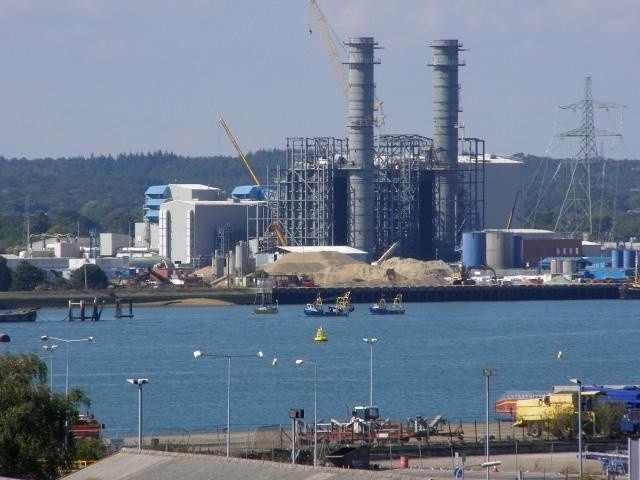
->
[253, 281, 411, 317]
[313, 322, 330, 342]
[69, 411, 105, 440]
[0, 305, 41, 322]
[490, 389, 553, 422]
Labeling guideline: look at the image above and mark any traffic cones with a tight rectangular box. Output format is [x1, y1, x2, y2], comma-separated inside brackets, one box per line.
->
[492, 457, 499, 472]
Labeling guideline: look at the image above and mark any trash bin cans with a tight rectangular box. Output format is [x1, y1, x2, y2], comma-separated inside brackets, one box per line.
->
[400, 456, 408, 469]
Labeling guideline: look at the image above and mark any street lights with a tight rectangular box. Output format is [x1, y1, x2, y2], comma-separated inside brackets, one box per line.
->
[295, 358, 320, 469]
[482, 366, 497, 479]
[40, 334, 96, 468]
[125, 376, 151, 453]
[455, 460, 501, 480]
[41, 343, 59, 404]
[192, 348, 267, 463]
[568, 377, 583, 480]
[361, 335, 378, 418]
[573, 410, 596, 440]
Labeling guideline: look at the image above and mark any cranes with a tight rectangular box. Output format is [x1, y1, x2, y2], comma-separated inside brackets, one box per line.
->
[307, 1, 388, 143]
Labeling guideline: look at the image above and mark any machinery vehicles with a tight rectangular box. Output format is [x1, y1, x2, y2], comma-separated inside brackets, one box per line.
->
[343, 403, 385, 428]
[509, 391, 591, 438]
[450, 260, 500, 285]
